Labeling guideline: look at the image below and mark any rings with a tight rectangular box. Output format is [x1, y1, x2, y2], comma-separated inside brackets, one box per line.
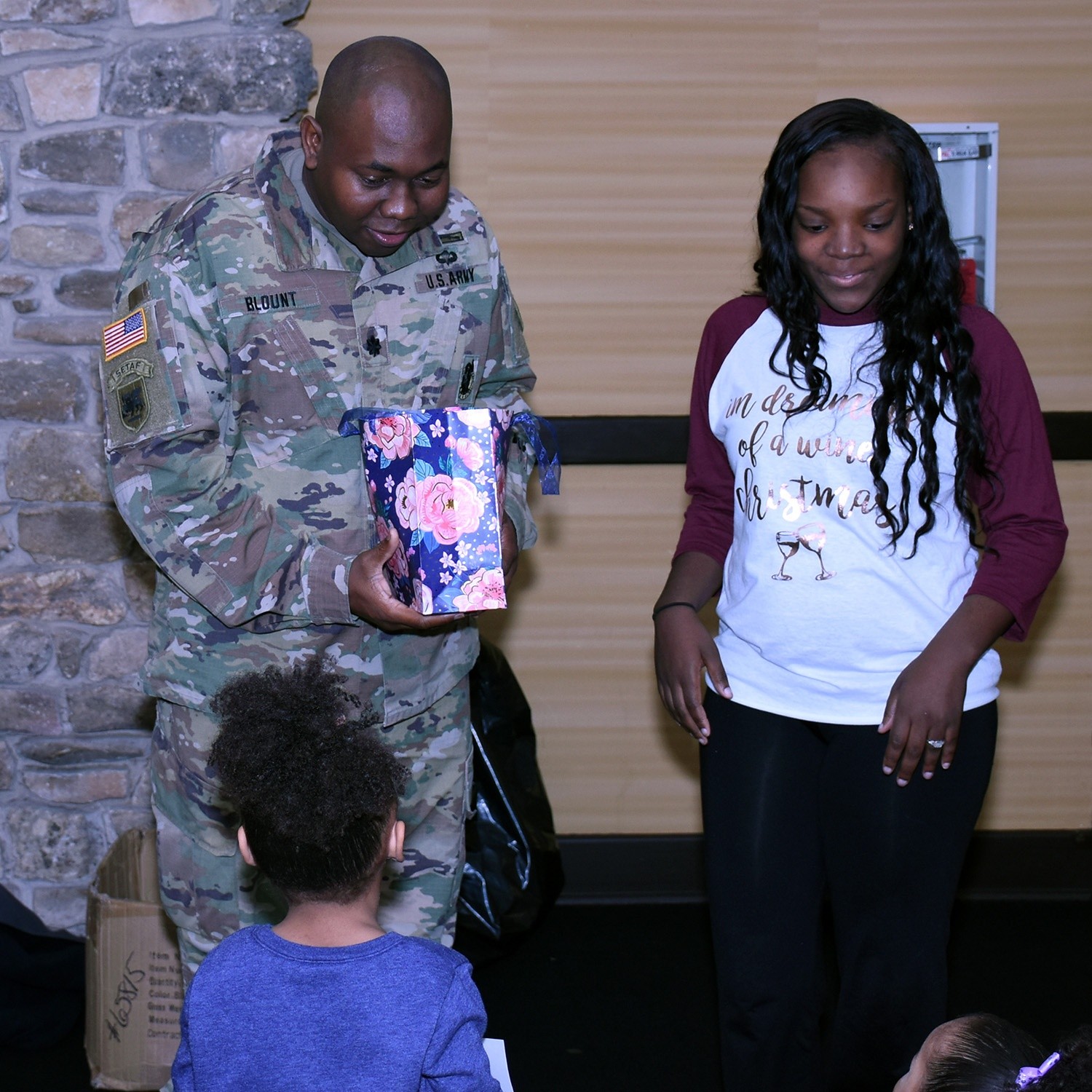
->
[925, 740, 946, 749]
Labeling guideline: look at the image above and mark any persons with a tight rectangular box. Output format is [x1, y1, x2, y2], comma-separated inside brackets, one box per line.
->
[890, 1011, 1092, 1092]
[95, 34, 542, 995]
[162, 663, 502, 1092]
[654, 99, 1069, 1091]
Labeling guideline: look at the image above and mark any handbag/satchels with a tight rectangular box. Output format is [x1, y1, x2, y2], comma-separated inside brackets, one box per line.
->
[337, 406, 562, 615]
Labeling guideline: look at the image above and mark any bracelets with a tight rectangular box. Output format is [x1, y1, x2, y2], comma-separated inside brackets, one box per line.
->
[651, 599, 696, 621]
